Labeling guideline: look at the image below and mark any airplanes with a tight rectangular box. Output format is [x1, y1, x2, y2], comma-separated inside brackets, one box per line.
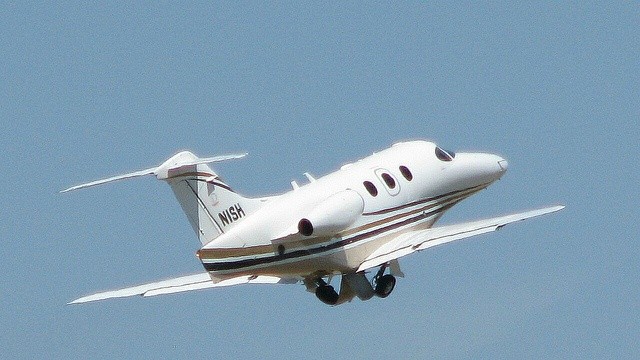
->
[60, 139, 564, 306]
